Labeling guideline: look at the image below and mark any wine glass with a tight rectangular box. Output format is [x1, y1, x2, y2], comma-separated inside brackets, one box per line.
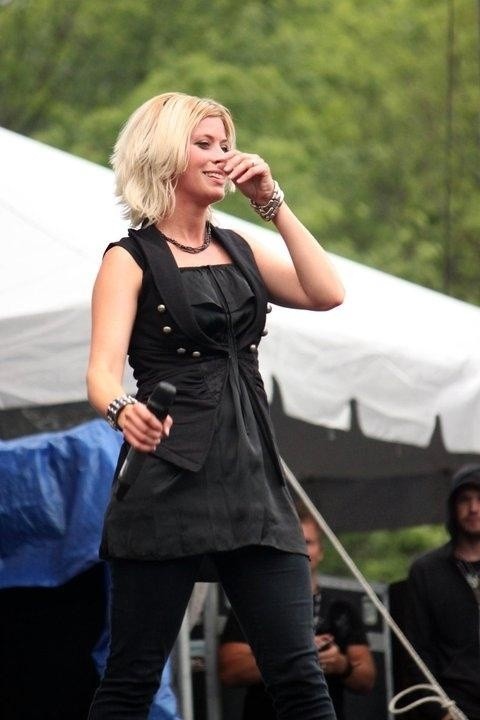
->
[251, 159, 255, 167]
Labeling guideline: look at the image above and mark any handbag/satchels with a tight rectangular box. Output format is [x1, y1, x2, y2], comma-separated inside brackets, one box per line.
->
[342, 657, 353, 680]
[105, 394, 137, 429]
[249, 180, 284, 223]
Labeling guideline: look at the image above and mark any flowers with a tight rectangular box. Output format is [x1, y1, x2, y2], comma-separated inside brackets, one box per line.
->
[113, 382, 176, 501]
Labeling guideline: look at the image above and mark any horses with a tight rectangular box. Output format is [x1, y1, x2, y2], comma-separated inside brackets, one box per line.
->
[317, 642, 332, 653]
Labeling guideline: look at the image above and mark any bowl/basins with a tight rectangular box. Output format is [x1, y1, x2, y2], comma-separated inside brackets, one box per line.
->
[164, 223, 211, 253]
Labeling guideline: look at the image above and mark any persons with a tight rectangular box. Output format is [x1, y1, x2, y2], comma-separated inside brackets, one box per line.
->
[399, 462, 480, 720]
[88, 93, 345, 720]
[216, 500, 377, 720]
